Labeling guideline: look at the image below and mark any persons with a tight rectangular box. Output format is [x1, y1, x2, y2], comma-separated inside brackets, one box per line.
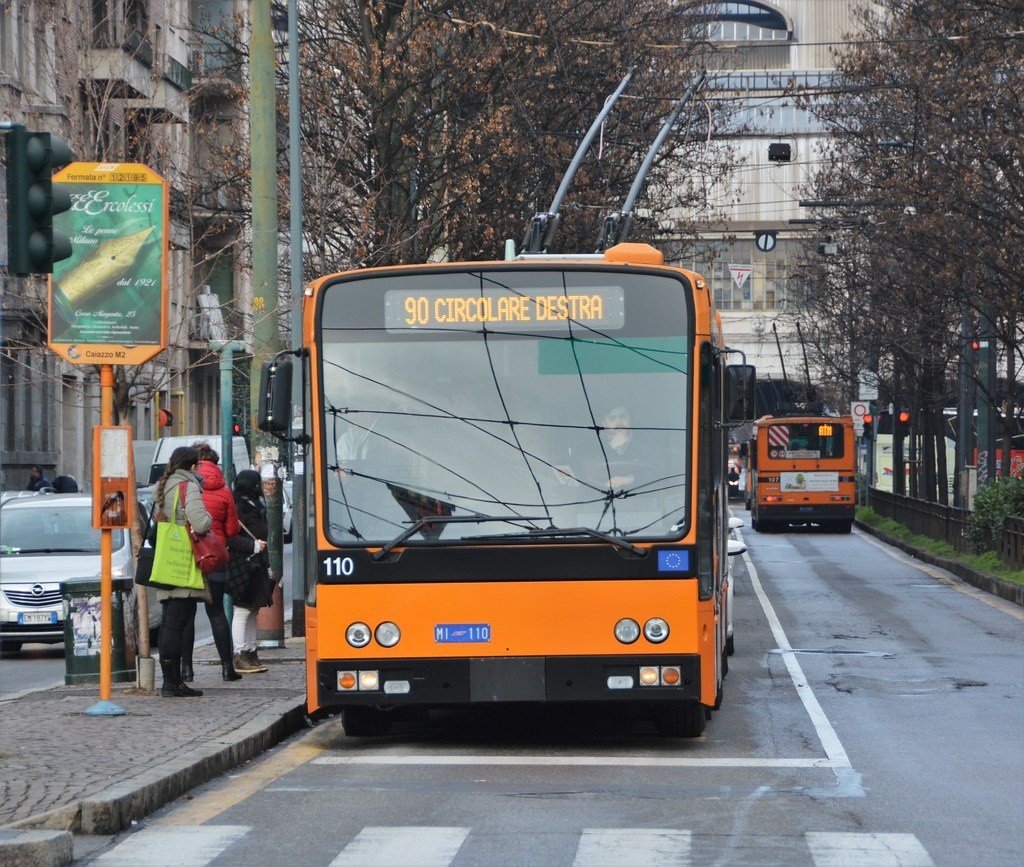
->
[182, 444, 243, 682]
[223, 468, 276, 672]
[26, 466, 51, 494]
[147, 446, 211, 697]
[52, 474, 78, 494]
[589, 410, 656, 490]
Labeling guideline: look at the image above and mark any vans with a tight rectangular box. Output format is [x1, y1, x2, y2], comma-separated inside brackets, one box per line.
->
[143, 434, 297, 552]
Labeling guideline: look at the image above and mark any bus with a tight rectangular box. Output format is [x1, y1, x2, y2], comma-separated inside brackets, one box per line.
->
[740, 413, 859, 534]
[257, 239, 759, 738]
[858, 405, 1024, 511]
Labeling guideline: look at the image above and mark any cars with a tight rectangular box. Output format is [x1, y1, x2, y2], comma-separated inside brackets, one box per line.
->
[727, 443, 742, 477]
[134, 483, 161, 524]
[0, 485, 57, 508]
[0, 492, 165, 653]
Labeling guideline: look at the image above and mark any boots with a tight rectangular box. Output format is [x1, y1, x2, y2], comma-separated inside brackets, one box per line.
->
[181, 662, 193, 681]
[247, 648, 268, 671]
[233, 651, 260, 672]
[219, 658, 243, 680]
[159, 658, 203, 696]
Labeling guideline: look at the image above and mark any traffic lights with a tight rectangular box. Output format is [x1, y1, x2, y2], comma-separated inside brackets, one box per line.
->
[863, 414, 877, 441]
[6, 128, 79, 278]
[899, 408, 911, 437]
[972, 341, 979, 350]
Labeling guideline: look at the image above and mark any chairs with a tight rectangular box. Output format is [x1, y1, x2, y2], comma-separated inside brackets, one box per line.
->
[5, 511, 46, 539]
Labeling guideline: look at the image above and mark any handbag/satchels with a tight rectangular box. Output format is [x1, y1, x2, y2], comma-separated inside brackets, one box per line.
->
[135, 499, 177, 591]
[149, 485, 205, 590]
[245, 545, 276, 608]
[178, 482, 230, 572]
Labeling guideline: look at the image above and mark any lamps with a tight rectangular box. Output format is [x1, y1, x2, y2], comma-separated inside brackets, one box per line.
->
[769, 143, 792, 161]
